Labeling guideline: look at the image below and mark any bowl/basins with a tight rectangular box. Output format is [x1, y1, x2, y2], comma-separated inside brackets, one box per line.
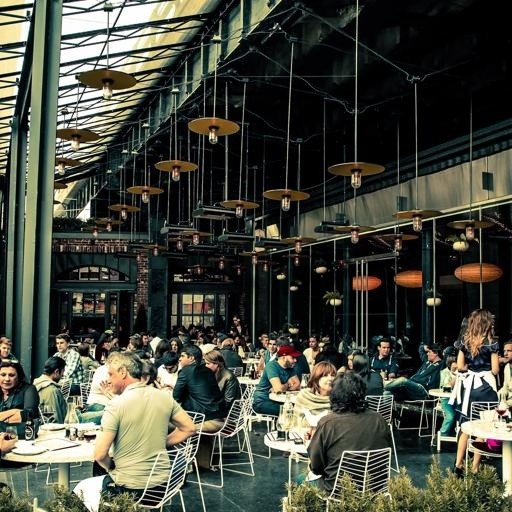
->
[84, 432, 97, 443]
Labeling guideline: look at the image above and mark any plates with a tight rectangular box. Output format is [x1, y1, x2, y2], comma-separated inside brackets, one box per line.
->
[13, 446, 49, 456]
[76, 423, 100, 431]
[40, 423, 64, 431]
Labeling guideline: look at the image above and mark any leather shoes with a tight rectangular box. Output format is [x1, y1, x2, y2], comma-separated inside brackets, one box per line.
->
[454, 467, 462, 475]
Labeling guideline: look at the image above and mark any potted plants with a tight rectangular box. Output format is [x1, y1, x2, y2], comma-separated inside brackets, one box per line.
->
[425, 288, 443, 307]
[323, 290, 345, 307]
[288, 319, 301, 334]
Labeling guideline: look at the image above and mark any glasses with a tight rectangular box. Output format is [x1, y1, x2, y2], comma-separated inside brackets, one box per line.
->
[1, 359, 19, 363]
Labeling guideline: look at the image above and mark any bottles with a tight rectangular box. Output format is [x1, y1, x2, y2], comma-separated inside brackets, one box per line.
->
[277, 396, 295, 434]
[25, 409, 36, 440]
[251, 363, 256, 380]
[64, 402, 80, 438]
[69, 427, 77, 442]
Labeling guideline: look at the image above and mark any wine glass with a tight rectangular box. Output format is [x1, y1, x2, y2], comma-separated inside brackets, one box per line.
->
[495, 403, 506, 421]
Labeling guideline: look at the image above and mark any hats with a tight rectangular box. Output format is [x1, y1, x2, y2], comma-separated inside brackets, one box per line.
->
[278, 345, 302, 358]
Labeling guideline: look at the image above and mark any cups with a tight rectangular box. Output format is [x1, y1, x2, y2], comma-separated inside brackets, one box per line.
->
[6, 426, 18, 442]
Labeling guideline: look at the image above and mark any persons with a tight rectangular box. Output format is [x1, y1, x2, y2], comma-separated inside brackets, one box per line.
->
[0, 299, 510, 512]
